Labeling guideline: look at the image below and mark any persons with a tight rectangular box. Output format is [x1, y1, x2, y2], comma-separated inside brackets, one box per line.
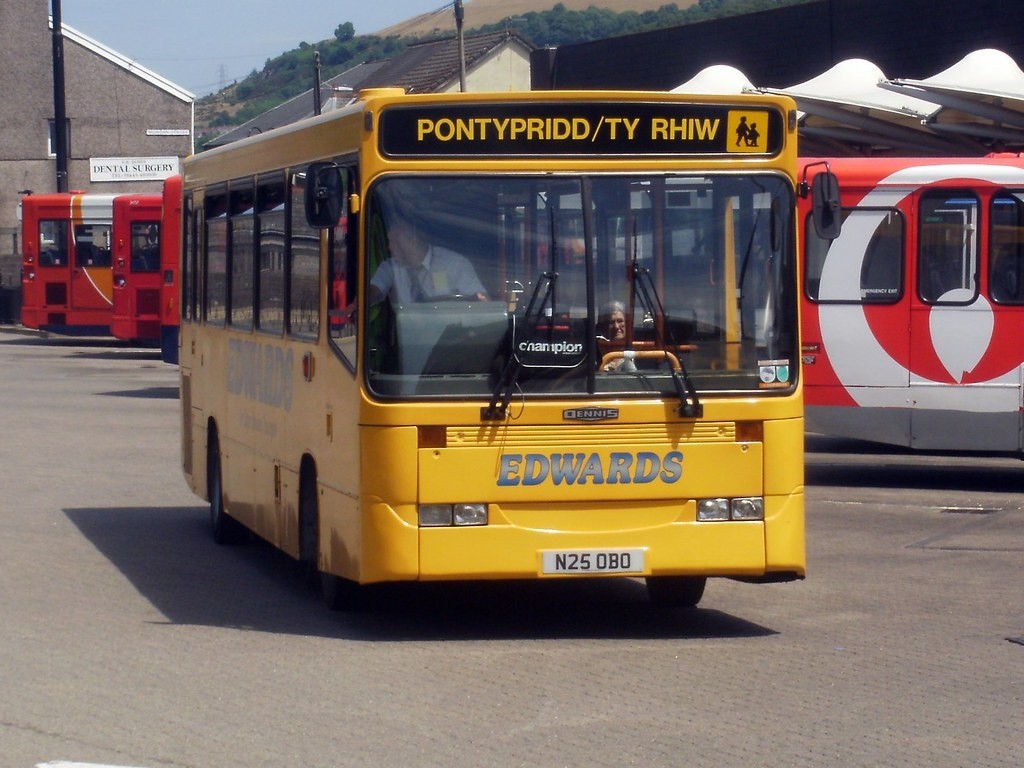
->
[574, 301, 658, 376]
[327, 215, 492, 318]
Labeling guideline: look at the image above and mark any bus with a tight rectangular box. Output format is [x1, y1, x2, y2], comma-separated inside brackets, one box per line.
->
[525, 154, 1024, 460]
[16, 185, 113, 341]
[180, 87, 810, 613]
[163, 172, 183, 364]
[113, 194, 161, 346]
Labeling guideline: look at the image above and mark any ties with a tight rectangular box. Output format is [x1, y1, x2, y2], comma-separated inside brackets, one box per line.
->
[405, 265, 426, 304]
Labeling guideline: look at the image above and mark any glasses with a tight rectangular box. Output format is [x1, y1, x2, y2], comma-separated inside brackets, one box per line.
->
[603, 319, 626, 328]
